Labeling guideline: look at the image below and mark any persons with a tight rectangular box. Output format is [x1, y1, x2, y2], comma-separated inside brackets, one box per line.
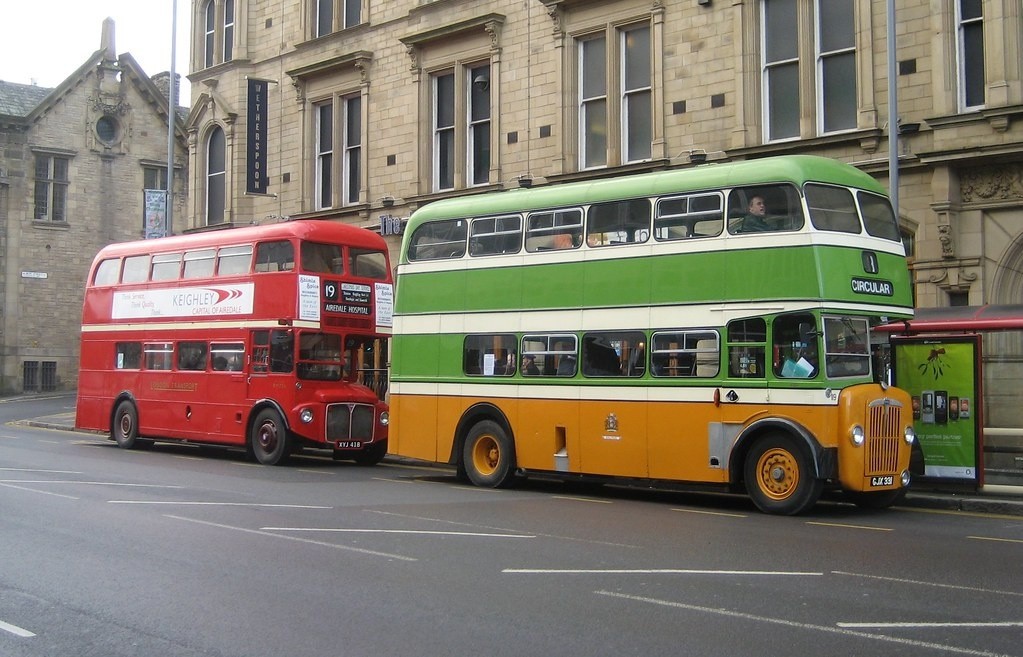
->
[740, 196, 780, 233]
[522, 352, 541, 375]
[503, 353, 518, 376]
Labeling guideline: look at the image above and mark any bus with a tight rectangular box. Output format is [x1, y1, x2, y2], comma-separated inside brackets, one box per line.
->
[384, 153, 917, 520]
[73, 220, 395, 468]
